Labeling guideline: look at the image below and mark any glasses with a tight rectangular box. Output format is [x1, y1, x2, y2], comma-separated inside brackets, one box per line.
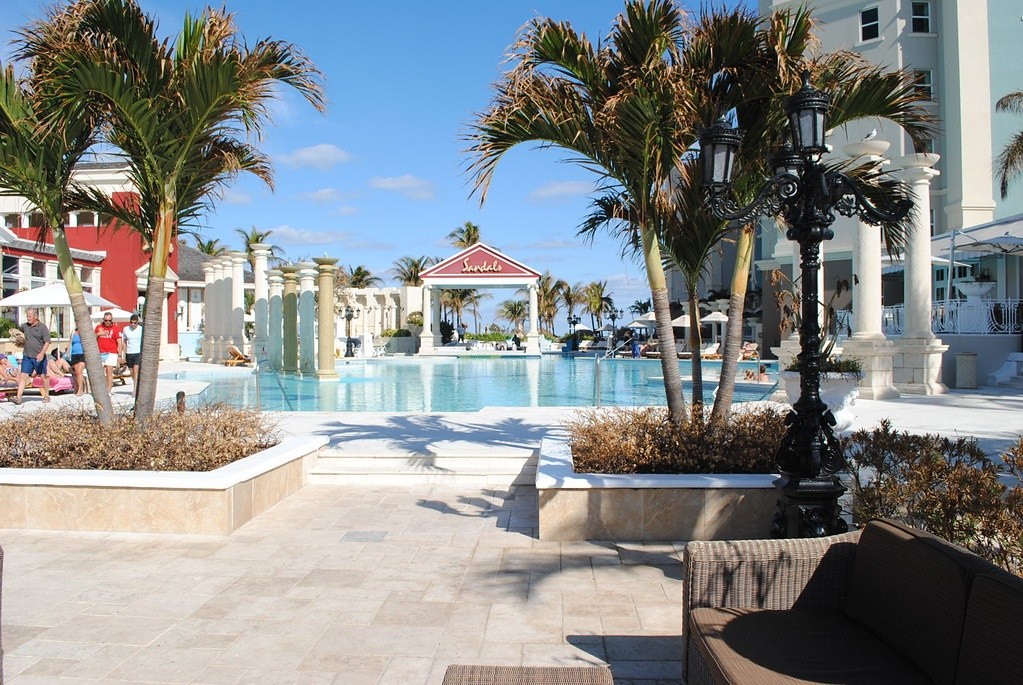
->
[130, 322, 137, 325]
[104, 318, 112, 323]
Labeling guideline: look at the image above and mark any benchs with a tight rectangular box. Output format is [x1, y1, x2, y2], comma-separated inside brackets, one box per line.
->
[681, 516, 1023, 685]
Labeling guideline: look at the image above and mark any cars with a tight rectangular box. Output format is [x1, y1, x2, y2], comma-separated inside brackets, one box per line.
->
[495, 337, 528, 350]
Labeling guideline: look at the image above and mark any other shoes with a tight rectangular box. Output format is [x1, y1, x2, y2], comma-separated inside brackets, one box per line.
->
[43, 395, 49, 403]
[10, 394, 21, 404]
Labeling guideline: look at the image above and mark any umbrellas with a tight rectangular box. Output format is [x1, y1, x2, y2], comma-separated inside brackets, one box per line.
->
[700, 312, 728, 343]
[568, 323, 591, 341]
[595, 323, 620, 336]
[0, 279, 122, 360]
[623, 320, 649, 328]
[636, 311, 656, 320]
[671, 315, 706, 338]
[89, 306, 142, 325]
[940, 231, 1023, 301]
[881, 251, 972, 267]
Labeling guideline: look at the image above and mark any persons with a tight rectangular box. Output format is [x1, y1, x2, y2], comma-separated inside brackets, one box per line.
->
[9, 305, 52, 405]
[745, 369, 754, 380]
[457, 325, 466, 343]
[495, 343, 506, 350]
[122, 314, 142, 395]
[623, 329, 641, 358]
[512, 334, 520, 346]
[639, 342, 649, 352]
[67, 327, 86, 395]
[51, 329, 74, 360]
[755, 364, 768, 381]
[0, 353, 32, 387]
[16, 358, 70, 377]
[95, 312, 122, 397]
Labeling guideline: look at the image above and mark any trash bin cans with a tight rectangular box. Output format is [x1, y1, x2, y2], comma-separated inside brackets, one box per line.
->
[954, 351, 978, 389]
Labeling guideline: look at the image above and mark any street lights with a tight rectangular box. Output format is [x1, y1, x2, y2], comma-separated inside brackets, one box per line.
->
[338, 304, 360, 357]
[698, 68, 913, 537]
[567, 314, 582, 350]
[604, 307, 625, 351]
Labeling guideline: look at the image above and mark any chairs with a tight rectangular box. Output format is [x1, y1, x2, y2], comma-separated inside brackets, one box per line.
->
[223, 346, 250, 367]
[578, 339, 759, 360]
[0, 353, 132, 403]
